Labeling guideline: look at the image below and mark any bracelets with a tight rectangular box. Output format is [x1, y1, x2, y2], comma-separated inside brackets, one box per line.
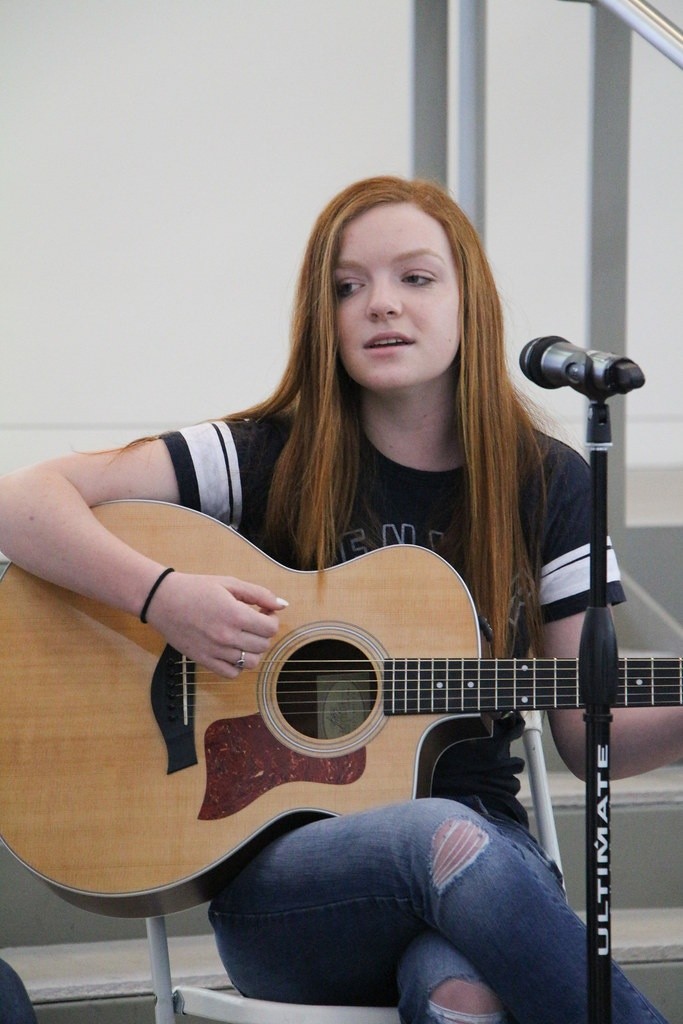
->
[140, 567, 174, 624]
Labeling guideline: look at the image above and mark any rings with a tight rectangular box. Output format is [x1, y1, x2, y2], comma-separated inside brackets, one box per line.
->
[236, 651, 245, 668]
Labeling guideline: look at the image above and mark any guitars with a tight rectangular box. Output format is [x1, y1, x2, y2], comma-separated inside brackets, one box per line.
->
[0, 498, 683, 918]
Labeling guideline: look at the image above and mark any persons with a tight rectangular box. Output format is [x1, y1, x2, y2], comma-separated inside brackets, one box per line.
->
[0, 176, 683, 1024]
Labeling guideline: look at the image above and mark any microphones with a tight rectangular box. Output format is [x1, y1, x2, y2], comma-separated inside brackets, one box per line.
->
[519, 335, 645, 395]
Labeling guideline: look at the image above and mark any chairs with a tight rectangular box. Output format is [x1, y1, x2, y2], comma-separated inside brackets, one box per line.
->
[142, 702, 568, 1024]
